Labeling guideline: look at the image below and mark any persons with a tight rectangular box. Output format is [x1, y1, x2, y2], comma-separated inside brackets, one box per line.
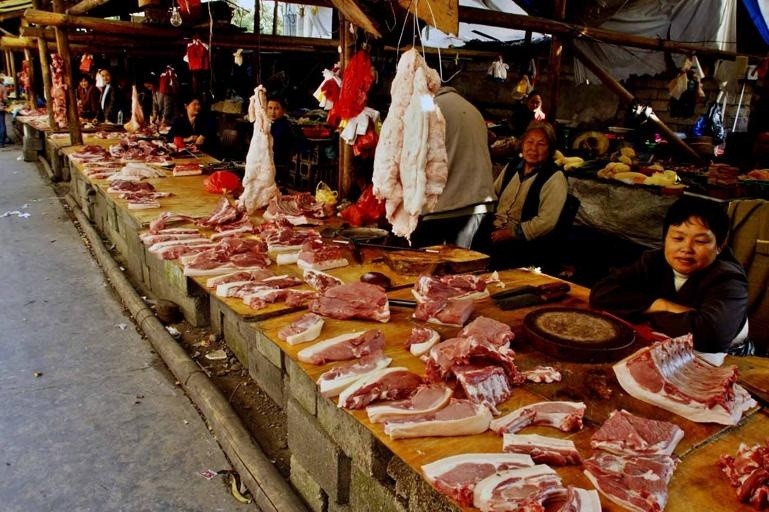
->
[423, 81, 499, 227]
[1, 60, 302, 193]
[472, 126, 568, 265]
[511, 92, 547, 124]
[590, 201, 755, 358]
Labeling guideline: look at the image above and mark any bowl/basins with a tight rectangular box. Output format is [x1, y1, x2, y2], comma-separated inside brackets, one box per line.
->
[303, 127, 331, 138]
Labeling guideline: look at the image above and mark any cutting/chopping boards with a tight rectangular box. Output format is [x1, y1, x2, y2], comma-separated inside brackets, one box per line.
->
[382, 245, 491, 278]
[522, 306, 638, 363]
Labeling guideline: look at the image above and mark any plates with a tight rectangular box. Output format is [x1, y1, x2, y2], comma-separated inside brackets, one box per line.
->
[338, 227, 389, 242]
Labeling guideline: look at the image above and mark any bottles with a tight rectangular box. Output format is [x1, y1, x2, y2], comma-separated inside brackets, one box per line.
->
[117, 110, 123, 126]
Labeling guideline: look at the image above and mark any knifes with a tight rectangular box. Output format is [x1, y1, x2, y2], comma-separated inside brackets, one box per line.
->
[489, 281, 571, 301]
